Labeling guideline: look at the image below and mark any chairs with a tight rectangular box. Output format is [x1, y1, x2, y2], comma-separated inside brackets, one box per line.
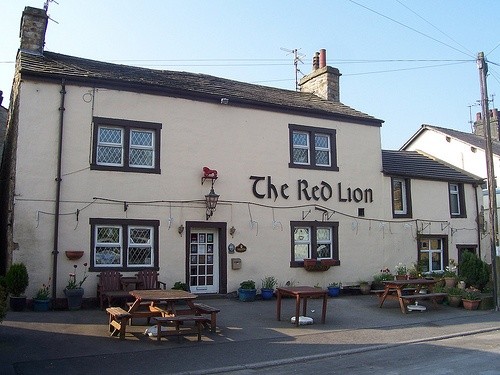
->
[96, 268, 167, 312]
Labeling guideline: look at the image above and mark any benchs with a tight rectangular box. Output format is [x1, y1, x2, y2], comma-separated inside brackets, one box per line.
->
[105, 301, 220, 345]
[370, 288, 417, 293]
[400, 292, 450, 300]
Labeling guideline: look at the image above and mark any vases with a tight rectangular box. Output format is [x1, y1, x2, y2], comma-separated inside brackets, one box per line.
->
[32, 297, 50, 312]
[62, 288, 84, 311]
[462, 299, 480, 310]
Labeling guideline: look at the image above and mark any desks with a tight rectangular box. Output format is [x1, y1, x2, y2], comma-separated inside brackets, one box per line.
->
[377, 278, 437, 314]
[276, 286, 327, 327]
[110, 289, 210, 337]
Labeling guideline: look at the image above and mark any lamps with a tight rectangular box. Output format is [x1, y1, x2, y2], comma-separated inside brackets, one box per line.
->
[204, 178, 220, 221]
[229, 226, 236, 235]
[179, 225, 184, 234]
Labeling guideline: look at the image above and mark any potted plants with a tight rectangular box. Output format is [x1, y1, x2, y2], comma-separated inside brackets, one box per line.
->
[360, 280, 371, 294]
[4, 262, 29, 311]
[371, 249, 493, 309]
[260, 276, 277, 300]
[172, 282, 191, 305]
[238, 280, 257, 301]
[327, 282, 341, 296]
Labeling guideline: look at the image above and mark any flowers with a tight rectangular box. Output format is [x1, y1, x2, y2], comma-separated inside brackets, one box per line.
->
[462, 286, 481, 301]
[36, 277, 51, 300]
[380, 268, 394, 281]
[64, 263, 89, 289]
